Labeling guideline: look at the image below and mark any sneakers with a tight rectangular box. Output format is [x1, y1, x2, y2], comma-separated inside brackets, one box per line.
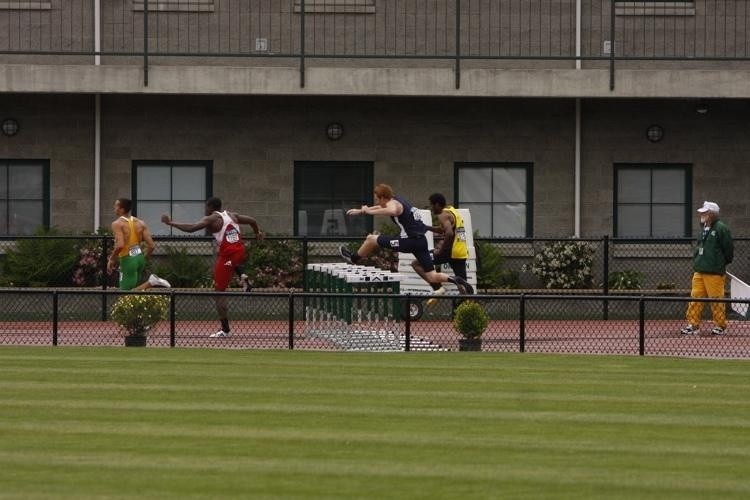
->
[209, 330, 232, 338]
[711, 326, 726, 335]
[427, 286, 446, 305]
[340, 246, 354, 265]
[148, 273, 170, 288]
[682, 325, 700, 335]
[455, 275, 474, 294]
[241, 274, 252, 291]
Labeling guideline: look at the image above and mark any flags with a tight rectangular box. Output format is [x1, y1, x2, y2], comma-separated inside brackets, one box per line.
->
[730, 276, 750, 317]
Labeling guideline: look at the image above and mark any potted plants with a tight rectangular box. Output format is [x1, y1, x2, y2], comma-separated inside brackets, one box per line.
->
[451, 298, 490, 351]
[111, 292, 171, 346]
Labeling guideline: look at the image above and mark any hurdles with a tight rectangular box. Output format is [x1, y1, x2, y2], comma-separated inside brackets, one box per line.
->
[304, 262, 451, 352]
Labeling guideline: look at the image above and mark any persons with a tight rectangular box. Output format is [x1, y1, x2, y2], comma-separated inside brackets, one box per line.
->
[680, 201, 733, 334]
[162, 197, 264, 338]
[339, 183, 474, 295]
[107, 199, 171, 290]
[412, 194, 469, 305]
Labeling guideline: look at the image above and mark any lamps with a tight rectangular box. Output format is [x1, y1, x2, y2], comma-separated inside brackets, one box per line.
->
[4, 120, 20, 139]
[647, 124, 664, 145]
[327, 125, 345, 142]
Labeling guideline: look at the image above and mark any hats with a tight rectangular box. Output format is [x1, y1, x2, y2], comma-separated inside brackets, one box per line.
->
[697, 200, 720, 213]
